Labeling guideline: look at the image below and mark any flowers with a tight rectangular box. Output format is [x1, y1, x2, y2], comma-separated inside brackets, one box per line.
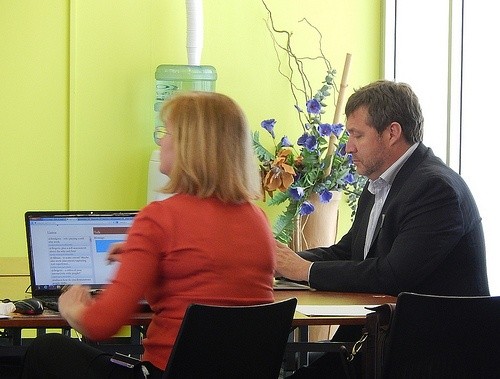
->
[251, 69, 371, 244]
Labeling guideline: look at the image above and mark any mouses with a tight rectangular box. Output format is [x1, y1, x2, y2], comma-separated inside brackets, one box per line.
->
[13, 299, 44, 316]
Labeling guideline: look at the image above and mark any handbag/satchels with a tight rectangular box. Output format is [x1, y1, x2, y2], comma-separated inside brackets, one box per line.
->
[346, 302, 397, 379]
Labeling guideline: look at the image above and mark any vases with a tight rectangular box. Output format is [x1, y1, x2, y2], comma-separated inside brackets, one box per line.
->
[300, 190, 339, 252]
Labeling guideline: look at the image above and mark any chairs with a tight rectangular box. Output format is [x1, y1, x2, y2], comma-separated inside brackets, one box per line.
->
[84, 299, 297, 379]
[340, 292, 500, 379]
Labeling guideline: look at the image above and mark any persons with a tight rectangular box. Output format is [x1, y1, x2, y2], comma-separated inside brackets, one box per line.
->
[20, 91, 278, 379]
[274, 80, 491, 379]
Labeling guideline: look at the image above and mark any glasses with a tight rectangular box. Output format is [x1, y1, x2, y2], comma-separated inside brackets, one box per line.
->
[153, 126, 168, 147]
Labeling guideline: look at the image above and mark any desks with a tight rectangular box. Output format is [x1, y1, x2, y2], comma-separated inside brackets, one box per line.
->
[0, 290, 398, 379]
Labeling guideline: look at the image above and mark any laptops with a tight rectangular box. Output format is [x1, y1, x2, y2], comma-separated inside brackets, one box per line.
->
[24, 211, 139, 313]
